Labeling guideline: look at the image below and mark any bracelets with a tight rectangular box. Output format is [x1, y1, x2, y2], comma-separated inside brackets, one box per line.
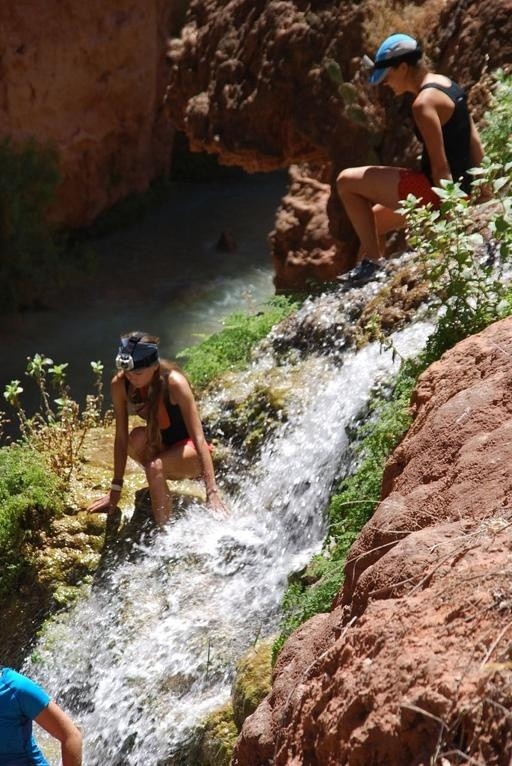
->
[206, 488, 218, 497]
[110, 477, 125, 493]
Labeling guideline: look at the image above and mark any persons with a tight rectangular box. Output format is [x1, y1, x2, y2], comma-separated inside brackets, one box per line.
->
[85, 331, 231, 535]
[1, 663, 85, 766]
[332, 33, 495, 288]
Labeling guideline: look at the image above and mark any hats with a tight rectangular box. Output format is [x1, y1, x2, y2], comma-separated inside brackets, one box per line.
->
[369, 33, 417, 87]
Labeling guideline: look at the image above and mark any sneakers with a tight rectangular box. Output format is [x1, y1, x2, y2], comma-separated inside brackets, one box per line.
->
[332, 257, 391, 285]
